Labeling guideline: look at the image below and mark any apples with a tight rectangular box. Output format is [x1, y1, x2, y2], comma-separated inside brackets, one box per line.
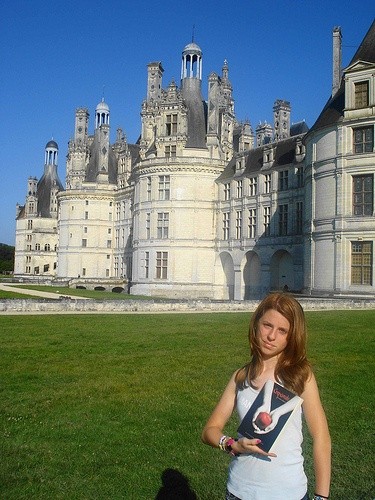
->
[255, 411, 272, 430]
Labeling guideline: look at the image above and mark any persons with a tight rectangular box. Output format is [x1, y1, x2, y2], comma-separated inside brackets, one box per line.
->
[200, 293, 331, 500]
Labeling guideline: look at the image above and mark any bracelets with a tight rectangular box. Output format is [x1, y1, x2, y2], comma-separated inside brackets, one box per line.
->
[218, 435, 240, 457]
[311, 493, 329, 500]
